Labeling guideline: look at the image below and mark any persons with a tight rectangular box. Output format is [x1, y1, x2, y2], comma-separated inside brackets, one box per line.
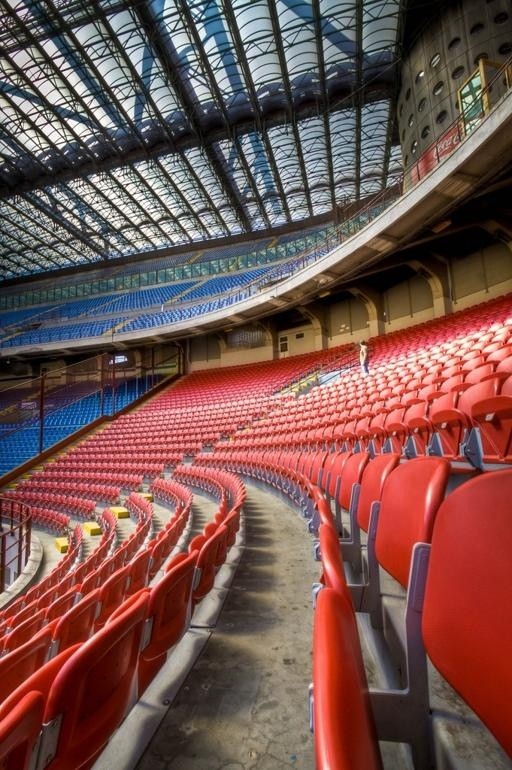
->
[359, 339, 369, 375]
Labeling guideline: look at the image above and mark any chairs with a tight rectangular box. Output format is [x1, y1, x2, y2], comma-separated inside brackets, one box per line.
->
[0, 223, 511, 769]
